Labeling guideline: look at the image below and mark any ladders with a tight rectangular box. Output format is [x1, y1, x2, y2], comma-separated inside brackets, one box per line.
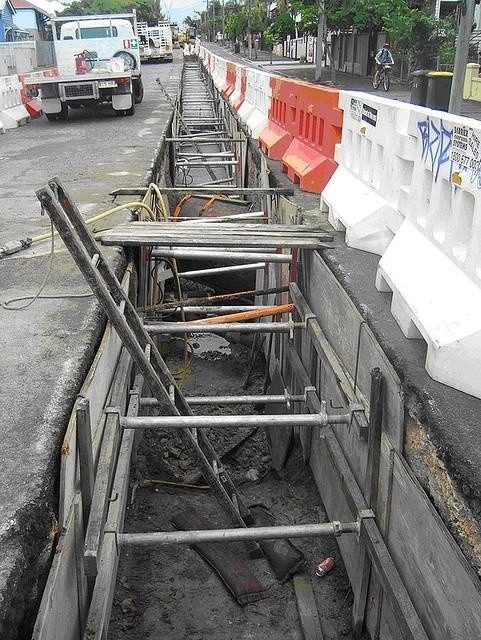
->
[156, 78, 220, 185]
[36, 176, 263, 560]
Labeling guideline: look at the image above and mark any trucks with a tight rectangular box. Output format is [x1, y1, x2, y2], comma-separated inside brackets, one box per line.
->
[24, 8, 145, 121]
[138, 20, 173, 64]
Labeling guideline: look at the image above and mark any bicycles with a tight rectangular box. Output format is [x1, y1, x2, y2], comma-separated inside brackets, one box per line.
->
[372, 62, 392, 92]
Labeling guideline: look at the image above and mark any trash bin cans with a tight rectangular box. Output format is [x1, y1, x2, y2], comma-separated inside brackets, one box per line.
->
[235, 44, 240, 53]
[410, 70, 453, 112]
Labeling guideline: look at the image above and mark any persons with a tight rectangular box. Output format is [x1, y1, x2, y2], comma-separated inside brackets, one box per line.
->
[372, 42, 394, 83]
